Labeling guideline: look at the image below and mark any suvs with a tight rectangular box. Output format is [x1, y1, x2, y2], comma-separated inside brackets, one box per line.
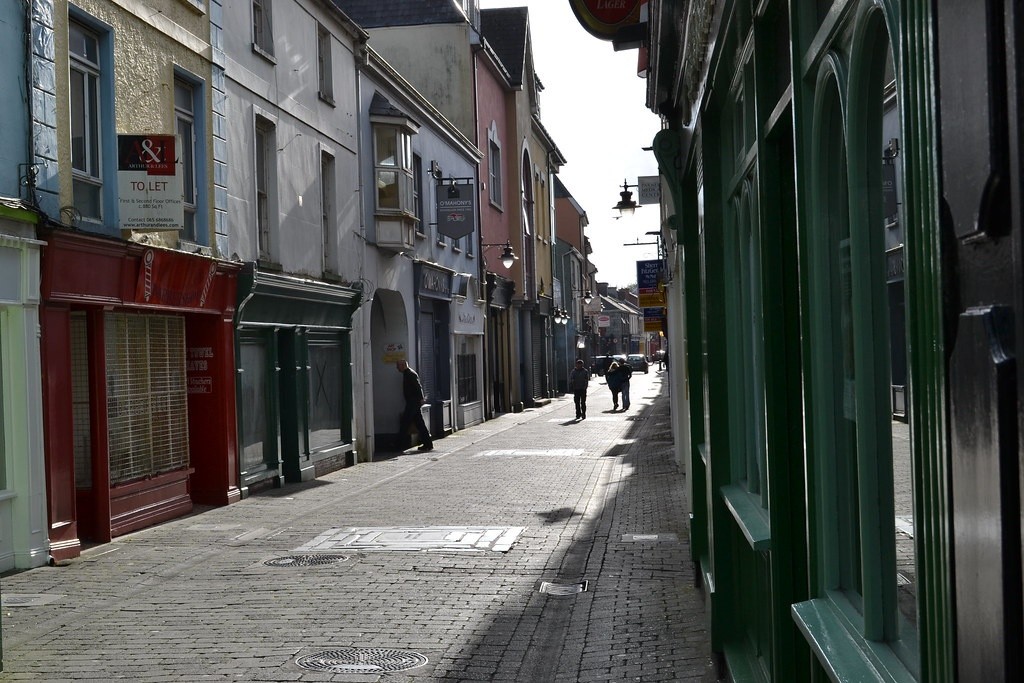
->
[591, 356, 620, 376]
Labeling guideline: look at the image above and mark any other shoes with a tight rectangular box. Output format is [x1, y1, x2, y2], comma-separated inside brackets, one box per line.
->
[389, 442, 397, 448]
[614, 404, 619, 409]
[418, 446, 433, 450]
[582, 413, 585, 419]
[576, 415, 580, 419]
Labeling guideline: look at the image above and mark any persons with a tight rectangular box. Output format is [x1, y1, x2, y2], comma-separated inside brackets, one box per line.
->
[391, 360, 433, 452]
[570, 360, 589, 419]
[602, 353, 632, 410]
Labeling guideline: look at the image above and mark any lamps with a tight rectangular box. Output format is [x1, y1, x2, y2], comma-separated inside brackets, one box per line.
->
[611, 177, 642, 218]
[560, 305, 571, 325]
[481, 238, 520, 269]
[551, 303, 562, 324]
[573, 288, 594, 305]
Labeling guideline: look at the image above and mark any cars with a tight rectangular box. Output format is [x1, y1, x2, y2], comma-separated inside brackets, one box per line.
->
[652, 350, 665, 361]
[626, 354, 648, 374]
